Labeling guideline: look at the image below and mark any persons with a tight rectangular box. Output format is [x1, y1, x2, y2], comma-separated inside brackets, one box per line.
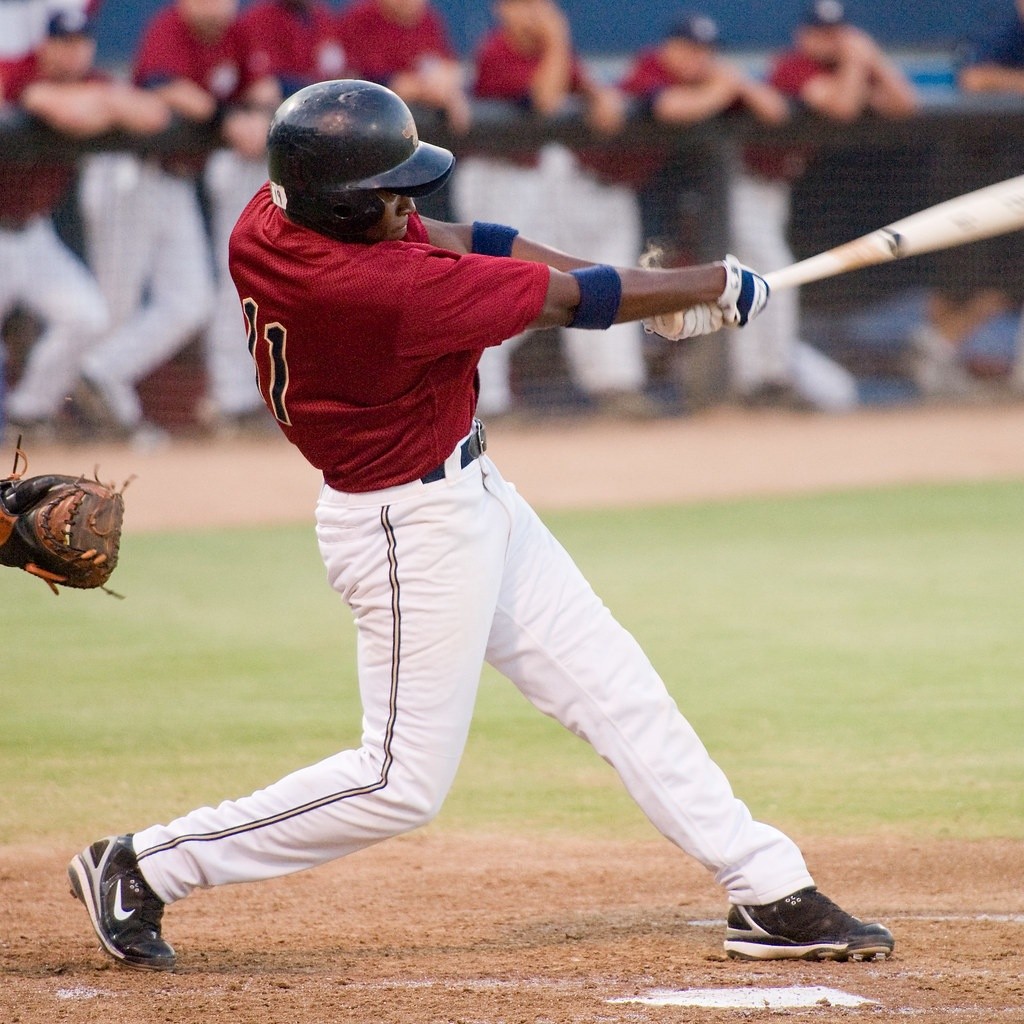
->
[0, 0, 1024, 968]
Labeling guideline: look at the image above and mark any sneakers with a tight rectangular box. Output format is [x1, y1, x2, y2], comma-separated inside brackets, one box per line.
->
[723, 885, 896, 962]
[65, 833, 177, 973]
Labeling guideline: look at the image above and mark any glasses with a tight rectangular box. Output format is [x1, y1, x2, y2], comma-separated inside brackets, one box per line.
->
[376, 193, 398, 204]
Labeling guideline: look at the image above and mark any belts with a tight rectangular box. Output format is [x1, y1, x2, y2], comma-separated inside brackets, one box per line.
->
[420, 417, 487, 484]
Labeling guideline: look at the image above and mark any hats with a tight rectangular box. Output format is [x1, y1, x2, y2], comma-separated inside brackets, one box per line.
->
[671, 15, 726, 47]
[800, 0, 849, 27]
[44, 8, 96, 37]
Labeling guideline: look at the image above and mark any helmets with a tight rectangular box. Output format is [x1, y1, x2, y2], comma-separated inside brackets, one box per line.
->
[266, 78, 456, 236]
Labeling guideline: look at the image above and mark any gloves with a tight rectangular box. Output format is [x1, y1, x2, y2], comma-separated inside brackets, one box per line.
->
[639, 300, 723, 341]
[712, 253, 771, 329]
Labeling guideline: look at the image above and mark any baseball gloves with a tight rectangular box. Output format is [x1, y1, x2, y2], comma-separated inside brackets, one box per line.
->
[0, 473, 125, 590]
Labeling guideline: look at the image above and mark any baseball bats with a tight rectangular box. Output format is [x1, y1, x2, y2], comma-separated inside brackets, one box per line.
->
[764, 173, 1024, 294]
